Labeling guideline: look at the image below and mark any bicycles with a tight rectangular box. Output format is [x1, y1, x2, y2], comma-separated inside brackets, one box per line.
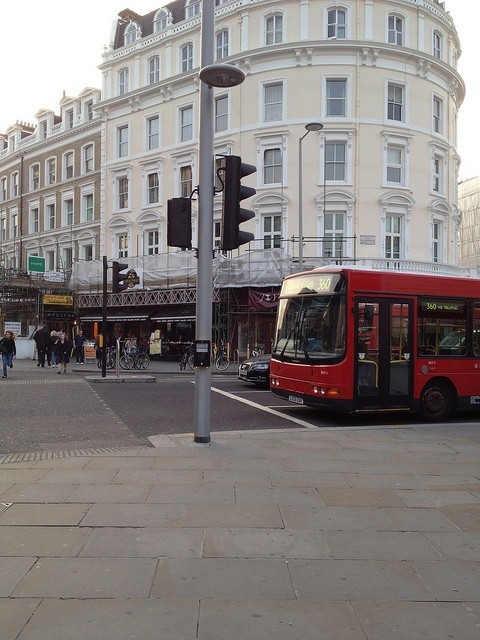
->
[215, 345, 230, 370]
[188, 346, 195, 372]
[119, 341, 134, 370]
[134, 342, 152, 370]
[97, 345, 116, 370]
[178, 347, 190, 371]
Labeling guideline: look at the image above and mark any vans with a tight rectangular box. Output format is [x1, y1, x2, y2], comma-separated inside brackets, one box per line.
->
[438, 329, 480, 349]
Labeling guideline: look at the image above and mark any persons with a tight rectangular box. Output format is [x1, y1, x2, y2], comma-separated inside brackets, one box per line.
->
[33, 325, 71, 374]
[72, 330, 86, 364]
[0, 330, 16, 377]
[92, 332, 116, 362]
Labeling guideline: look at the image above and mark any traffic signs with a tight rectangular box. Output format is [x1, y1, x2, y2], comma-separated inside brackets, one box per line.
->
[27, 254, 46, 273]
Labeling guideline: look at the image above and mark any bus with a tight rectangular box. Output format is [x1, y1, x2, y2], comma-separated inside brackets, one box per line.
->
[269, 265, 480, 422]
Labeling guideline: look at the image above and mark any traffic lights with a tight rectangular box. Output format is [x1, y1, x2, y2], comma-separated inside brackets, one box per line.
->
[112, 261, 128, 293]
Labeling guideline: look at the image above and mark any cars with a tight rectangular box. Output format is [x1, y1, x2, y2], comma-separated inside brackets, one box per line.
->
[238, 354, 272, 386]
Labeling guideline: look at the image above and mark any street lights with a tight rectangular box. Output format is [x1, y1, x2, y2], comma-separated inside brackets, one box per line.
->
[193, 0, 245, 442]
[220, 155, 257, 250]
[298, 122, 323, 272]
